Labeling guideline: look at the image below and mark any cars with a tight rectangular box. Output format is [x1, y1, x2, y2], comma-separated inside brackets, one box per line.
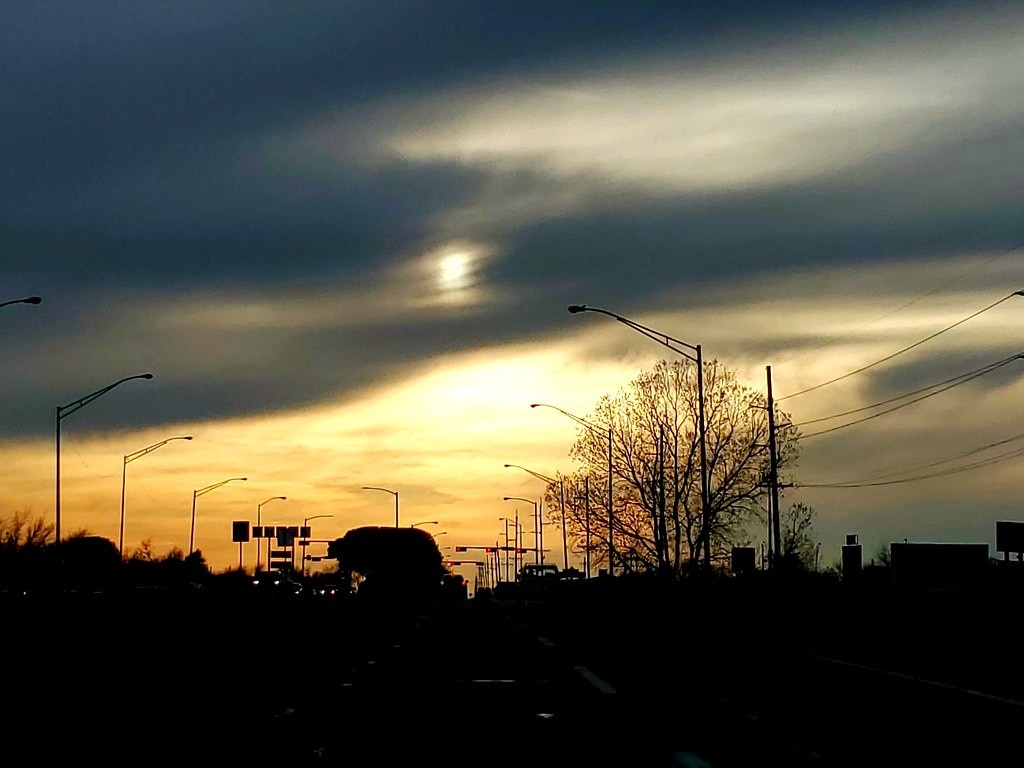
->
[517, 563, 557, 582]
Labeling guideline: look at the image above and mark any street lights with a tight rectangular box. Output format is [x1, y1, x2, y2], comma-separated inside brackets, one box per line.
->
[120, 436, 195, 558]
[410, 520, 439, 530]
[190, 476, 250, 556]
[256, 496, 287, 573]
[502, 463, 569, 572]
[302, 514, 335, 575]
[529, 402, 616, 577]
[359, 486, 399, 527]
[567, 302, 712, 566]
[498, 516, 523, 569]
[502, 496, 539, 564]
[52, 373, 155, 543]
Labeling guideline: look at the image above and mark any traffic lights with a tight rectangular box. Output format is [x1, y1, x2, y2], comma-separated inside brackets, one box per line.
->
[486, 548, 497, 553]
[516, 548, 527, 554]
[450, 562, 463, 565]
[456, 547, 467, 552]
